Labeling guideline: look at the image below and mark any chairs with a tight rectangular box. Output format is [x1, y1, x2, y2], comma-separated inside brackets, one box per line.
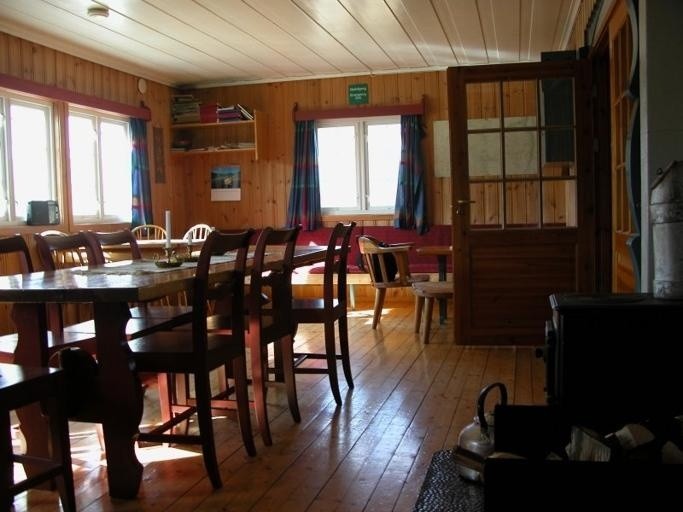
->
[36, 229, 169, 404]
[1, 364, 78, 512]
[250, 222, 355, 404]
[70, 223, 219, 262]
[158, 224, 305, 453]
[94, 228, 197, 334]
[1, 227, 99, 412]
[104, 226, 254, 489]
[358, 234, 429, 329]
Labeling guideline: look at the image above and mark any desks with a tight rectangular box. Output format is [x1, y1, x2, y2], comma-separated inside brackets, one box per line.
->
[416, 244, 453, 325]
[0, 243, 351, 439]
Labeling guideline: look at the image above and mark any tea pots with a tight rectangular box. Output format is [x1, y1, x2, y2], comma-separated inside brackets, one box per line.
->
[457, 382, 507, 483]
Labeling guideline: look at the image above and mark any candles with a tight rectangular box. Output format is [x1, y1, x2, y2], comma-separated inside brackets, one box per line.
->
[165, 210, 171, 248]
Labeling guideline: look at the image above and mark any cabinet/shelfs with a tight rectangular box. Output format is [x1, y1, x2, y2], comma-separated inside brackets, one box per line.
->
[170, 108, 261, 161]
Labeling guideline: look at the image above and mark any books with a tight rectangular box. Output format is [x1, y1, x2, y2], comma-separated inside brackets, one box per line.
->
[170, 89, 254, 150]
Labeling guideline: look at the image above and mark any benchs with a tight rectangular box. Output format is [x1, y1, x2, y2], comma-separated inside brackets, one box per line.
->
[183, 223, 566, 311]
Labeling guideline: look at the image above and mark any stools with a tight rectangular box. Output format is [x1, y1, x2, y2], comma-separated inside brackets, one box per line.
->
[410, 281, 454, 345]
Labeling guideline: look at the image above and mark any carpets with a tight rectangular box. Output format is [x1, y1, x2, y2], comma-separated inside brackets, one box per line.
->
[411, 449, 485, 512]
